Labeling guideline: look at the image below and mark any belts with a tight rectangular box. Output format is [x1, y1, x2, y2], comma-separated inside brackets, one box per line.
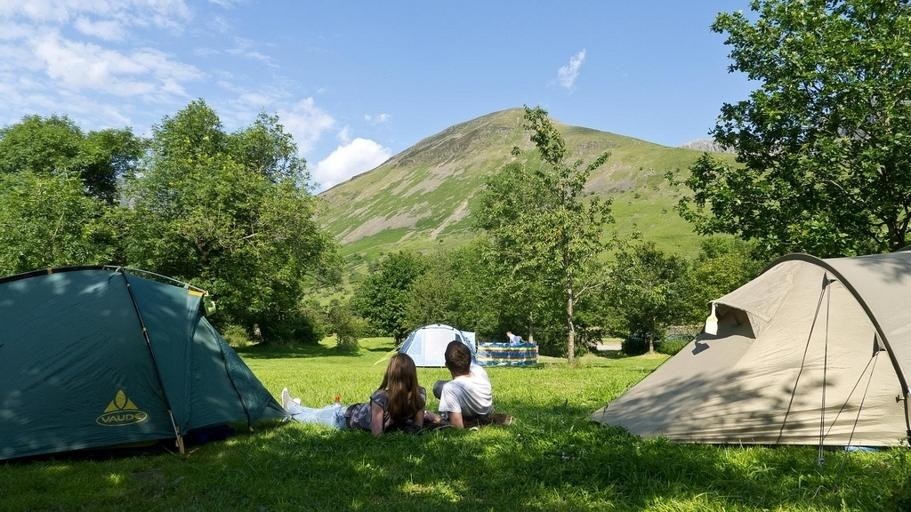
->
[344, 403, 360, 429]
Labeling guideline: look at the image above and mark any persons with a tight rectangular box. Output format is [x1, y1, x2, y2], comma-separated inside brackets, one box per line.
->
[280, 353, 429, 438]
[424, 339, 498, 430]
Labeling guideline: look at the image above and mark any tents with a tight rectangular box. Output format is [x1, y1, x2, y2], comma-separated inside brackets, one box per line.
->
[584, 244, 911, 449]
[398, 322, 486, 370]
[0, 264, 294, 467]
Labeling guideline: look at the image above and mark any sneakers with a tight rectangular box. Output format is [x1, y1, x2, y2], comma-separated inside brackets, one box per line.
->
[281, 388, 301, 414]
